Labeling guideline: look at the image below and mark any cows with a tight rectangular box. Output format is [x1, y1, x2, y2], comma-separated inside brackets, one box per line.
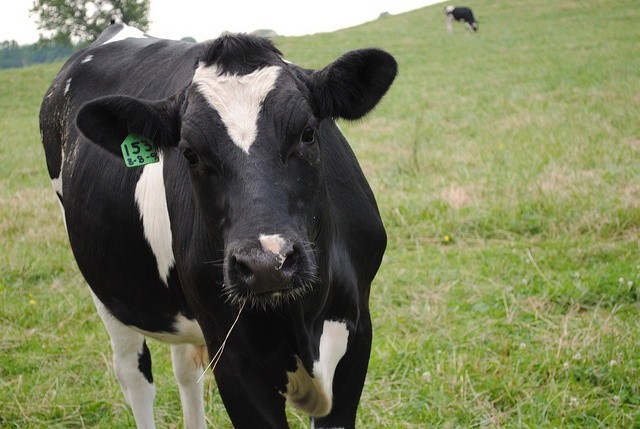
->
[39, 20, 398, 429]
[445, 6, 480, 34]
